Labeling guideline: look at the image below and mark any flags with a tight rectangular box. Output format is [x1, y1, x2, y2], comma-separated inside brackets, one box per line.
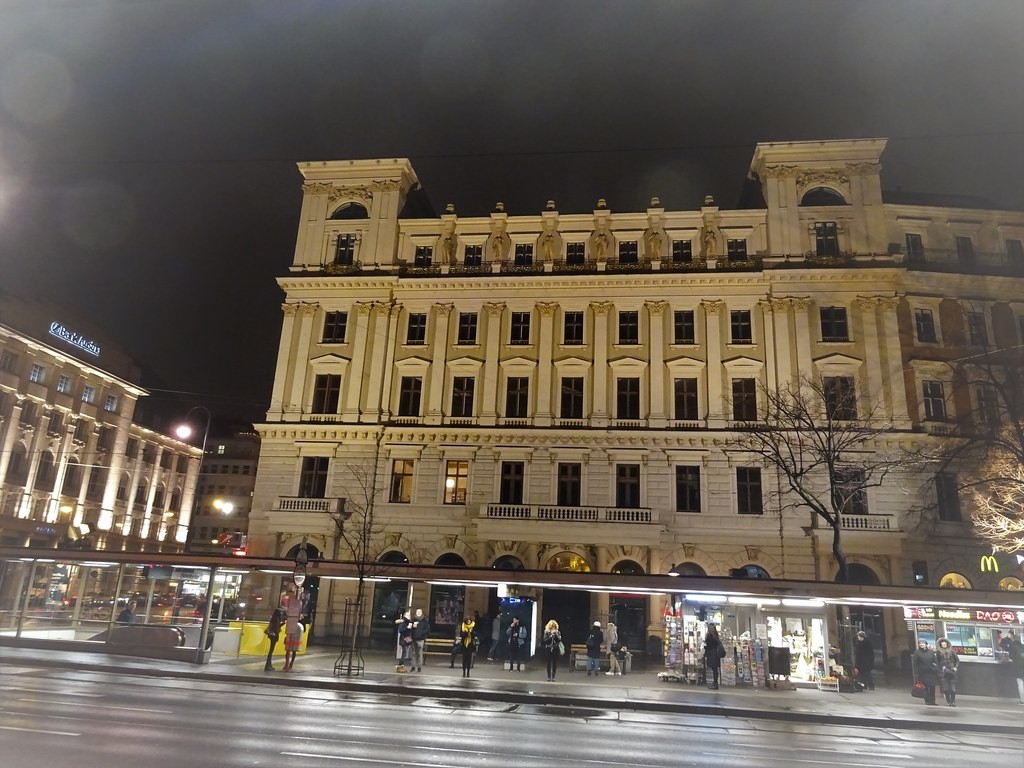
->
[661, 604, 673, 626]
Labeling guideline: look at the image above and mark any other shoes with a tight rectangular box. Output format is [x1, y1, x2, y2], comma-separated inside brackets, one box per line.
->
[410, 667, 415, 672]
[595, 671, 599, 675]
[947, 701, 956, 706]
[1016, 700, 1024, 705]
[615, 672, 621, 675]
[509, 668, 513, 672]
[517, 668, 520, 672]
[588, 670, 592, 675]
[417, 667, 421, 672]
[552, 677, 556, 681]
[605, 671, 614, 675]
[708, 682, 718, 689]
[265, 666, 275, 671]
[547, 677, 551, 681]
[926, 701, 937, 705]
[487, 657, 498, 661]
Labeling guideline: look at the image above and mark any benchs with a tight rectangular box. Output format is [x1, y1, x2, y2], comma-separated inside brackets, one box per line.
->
[423, 639, 479, 668]
[569, 644, 627, 675]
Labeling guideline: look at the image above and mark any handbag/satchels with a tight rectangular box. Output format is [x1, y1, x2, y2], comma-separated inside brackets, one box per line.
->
[911, 675, 928, 697]
[558, 641, 565, 655]
[264, 627, 276, 636]
[611, 642, 622, 652]
[404, 636, 413, 643]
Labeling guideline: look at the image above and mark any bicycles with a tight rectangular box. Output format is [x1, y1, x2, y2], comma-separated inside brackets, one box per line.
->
[78, 605, 111, 620]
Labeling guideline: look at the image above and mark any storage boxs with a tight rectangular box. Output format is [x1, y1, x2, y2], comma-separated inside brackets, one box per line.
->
[817, 678, 840, 692]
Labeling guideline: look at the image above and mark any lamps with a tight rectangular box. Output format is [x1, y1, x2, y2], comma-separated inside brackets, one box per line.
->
[668, 563, 680, 577]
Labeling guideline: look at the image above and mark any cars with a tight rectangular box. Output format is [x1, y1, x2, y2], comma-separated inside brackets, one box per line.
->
[178, 592, 239, 620]
[74, 588, 175, 608]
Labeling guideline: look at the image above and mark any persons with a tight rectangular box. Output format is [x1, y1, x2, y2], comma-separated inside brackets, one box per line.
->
[450, 616, 478, 677]
[912, 637, 941, 705]
[605, 623, 622, 676]
[282, 618, 304, 670]
[487, 614, 501, 662]
[117, 600, 137, 627]
[854, 631, 876, 690]
[704, 623, 721, 690]
[265, 608, 288, 671]
[931, 637, 959, 707]
[543, 620, 562, 682]
[996, 629, 1024, 707]
[409, 608, 430, 673]
[395, 610, 412, 673]
[193, 593, 240, 623]
[585, 620, 603, 675]
[506, 617, 527, 672]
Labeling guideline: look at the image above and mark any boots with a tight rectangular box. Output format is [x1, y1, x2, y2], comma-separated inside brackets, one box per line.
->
[467, 667, 470, 676]
[395, 665, 407, 673]
[463, 666, 465, 677]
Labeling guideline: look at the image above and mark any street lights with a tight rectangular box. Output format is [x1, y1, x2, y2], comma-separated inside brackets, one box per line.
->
[199, 499, 232, 650]
[170, 406, 211, 625]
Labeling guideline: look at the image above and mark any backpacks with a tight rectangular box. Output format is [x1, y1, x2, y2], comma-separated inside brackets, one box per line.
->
[586, 631, 601, 647]
[712, 633, 726, 658]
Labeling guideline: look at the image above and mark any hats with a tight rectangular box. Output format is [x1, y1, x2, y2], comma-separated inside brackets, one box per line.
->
[857, 631, 866, 637]
[594, 621, 601, 626]
[919, 638, 928, 644]
